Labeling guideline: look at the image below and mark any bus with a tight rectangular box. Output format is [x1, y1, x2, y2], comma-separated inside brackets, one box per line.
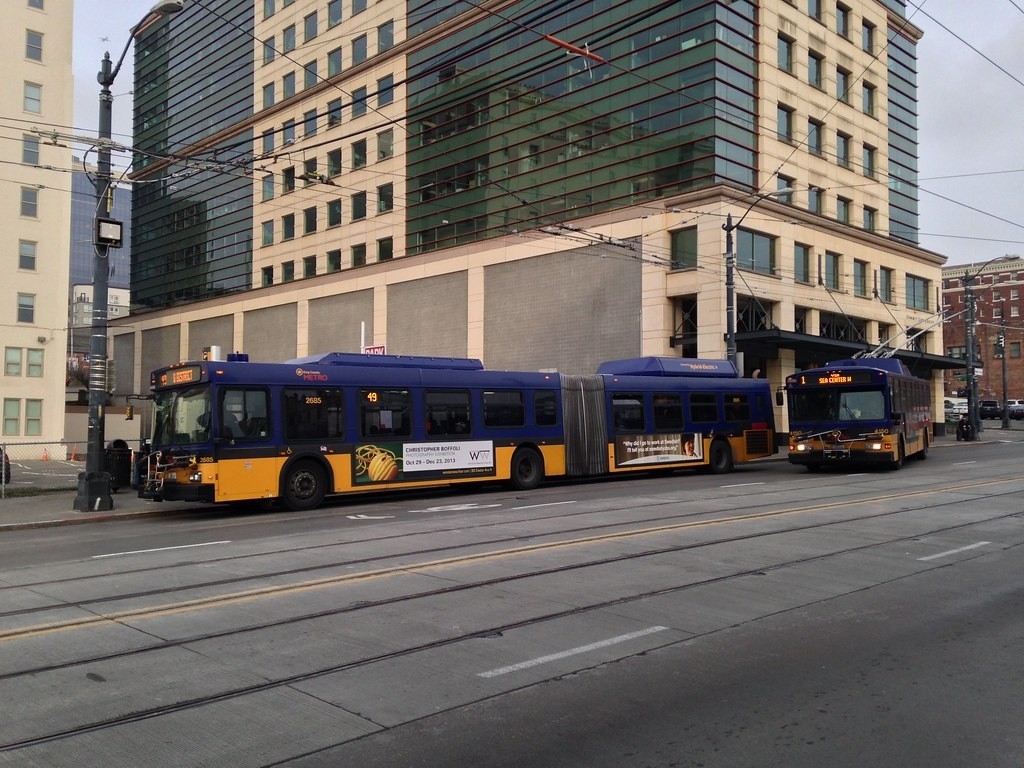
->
[774, 358, 933, 475]
[125, 350, 778, 509]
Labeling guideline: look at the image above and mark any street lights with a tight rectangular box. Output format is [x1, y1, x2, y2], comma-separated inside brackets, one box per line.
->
[721, 185, 796, 369]
[959, 253, 1019, 439]
[73, 0, 185, 512]
[970, 279, 1018, 432]
[997, 297, 1012, 431]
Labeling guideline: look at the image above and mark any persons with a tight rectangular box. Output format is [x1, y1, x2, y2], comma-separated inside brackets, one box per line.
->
[959, 413, 971, 439]
[196, 409, 471, 442]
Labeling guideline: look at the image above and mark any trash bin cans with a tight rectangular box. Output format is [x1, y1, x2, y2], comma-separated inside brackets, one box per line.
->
[104, 439, 132, 494]
